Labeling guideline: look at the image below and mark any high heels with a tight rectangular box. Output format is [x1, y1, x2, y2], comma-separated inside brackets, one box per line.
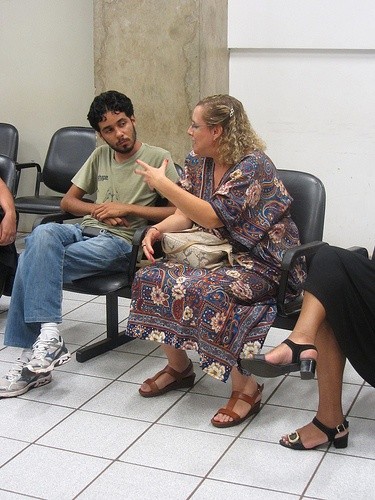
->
[209, 382, 265, 429]
[235, 338, 320, 383]
[277, 413, 351, 452]
[138, 358, 198, 399]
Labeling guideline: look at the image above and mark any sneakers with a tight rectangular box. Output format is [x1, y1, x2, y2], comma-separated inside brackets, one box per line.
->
[21, 332, 72, 380]
[0, 353, 54, 399]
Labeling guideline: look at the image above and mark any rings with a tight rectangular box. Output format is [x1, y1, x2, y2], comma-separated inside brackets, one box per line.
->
[142, 245, 146, 248]
[10, 237, 14, 239]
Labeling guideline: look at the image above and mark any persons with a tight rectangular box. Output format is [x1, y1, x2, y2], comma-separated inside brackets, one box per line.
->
[125, 93, 307, 428]
[0, 178, 19, 297]
[0, 89, 183, 396]
[240, 246, 375, 451]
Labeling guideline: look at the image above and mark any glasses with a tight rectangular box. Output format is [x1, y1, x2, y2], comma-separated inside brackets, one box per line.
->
[189, 121, 208, 131]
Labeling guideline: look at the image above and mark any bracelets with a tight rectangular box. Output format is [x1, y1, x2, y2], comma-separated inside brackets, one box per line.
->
[146, 227, 158, 234]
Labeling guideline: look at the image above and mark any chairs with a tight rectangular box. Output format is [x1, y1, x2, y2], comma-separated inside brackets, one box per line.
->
[14, 126, 97, 237]
[0, 122, 19, 239]
[0, 153, 21, 298]
[270, 167, 327, 332]
[31, 160, 185, 363]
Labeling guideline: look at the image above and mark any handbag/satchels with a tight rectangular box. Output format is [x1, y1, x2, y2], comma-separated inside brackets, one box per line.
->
[160, 223, 239, 272]
